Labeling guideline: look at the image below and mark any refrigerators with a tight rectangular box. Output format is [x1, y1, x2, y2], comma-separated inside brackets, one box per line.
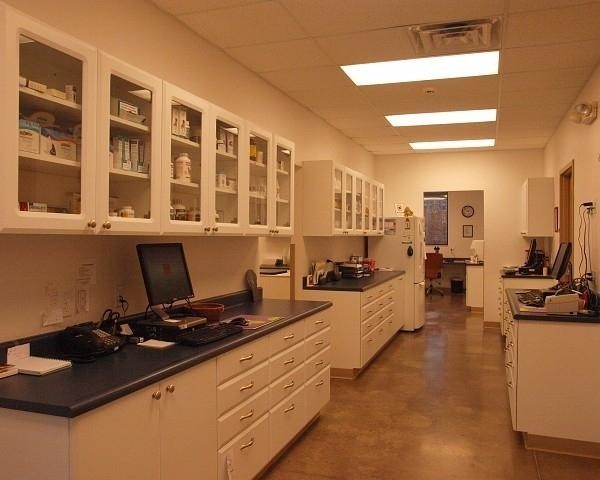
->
[367, 216, 426, 332]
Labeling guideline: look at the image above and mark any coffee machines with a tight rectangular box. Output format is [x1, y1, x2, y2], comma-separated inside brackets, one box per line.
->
[469, 240, 484, 263]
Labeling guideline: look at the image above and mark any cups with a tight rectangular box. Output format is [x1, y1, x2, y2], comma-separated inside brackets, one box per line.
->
[543, 267, 548, 276]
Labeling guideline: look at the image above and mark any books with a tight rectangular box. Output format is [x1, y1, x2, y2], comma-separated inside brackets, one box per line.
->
[0, 355, 72, 379]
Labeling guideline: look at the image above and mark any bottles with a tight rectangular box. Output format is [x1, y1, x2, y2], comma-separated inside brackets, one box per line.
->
[170, 152, 192, 181]
[109, 206, 137, 217]
[475, 257, 478, 265]
[170, 198, 201, 221]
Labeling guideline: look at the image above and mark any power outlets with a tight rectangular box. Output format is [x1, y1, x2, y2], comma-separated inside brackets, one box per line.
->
[583, 201, 597, 214]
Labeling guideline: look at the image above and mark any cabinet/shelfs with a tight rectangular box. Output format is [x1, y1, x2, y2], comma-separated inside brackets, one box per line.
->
[301, 274, 406, 380]
[301, 159, 385, 237]
[521, 177, 555, 237]
[463, 264, 484, 312]
[497, 273, 600, 460]
[260, 273, 289, 302]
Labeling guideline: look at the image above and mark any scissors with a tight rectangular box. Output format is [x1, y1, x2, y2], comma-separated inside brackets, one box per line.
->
[103, 309, 120, 323]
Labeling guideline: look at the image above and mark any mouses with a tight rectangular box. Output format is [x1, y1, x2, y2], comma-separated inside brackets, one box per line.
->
[230, 318, 246, 326]
[526, 302, 537, 307]
[505, 270, 516, 274]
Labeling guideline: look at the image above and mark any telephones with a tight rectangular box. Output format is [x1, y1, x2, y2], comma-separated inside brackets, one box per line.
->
[64, 325, 122, 357]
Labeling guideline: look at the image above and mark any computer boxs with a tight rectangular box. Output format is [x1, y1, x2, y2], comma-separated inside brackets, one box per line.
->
[541, 289, 575, 304]
[134, 316, 208, 343]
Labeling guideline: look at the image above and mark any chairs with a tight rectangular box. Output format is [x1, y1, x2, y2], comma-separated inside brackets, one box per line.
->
[424, 253, 444, 299]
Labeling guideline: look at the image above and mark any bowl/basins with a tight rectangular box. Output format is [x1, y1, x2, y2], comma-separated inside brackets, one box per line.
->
[186, 302, 225, 320]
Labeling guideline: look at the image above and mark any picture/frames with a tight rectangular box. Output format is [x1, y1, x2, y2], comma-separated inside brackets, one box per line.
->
[463, 225, 473, 237]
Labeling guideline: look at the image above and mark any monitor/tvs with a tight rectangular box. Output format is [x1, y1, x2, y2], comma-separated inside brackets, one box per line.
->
[550, 242, 572, 280]
[523, 239, 537, 268]
[136, 241, 195, 323]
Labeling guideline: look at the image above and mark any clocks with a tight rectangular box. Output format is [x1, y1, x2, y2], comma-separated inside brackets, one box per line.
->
[462, 205, 474, 218]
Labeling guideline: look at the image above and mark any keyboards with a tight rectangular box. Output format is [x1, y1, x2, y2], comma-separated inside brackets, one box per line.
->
[519, 293, 543, 304]
[175, 322, 243, 347]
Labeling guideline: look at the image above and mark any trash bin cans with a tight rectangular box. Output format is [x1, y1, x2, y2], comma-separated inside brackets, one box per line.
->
[451, 277, 463, 292]
